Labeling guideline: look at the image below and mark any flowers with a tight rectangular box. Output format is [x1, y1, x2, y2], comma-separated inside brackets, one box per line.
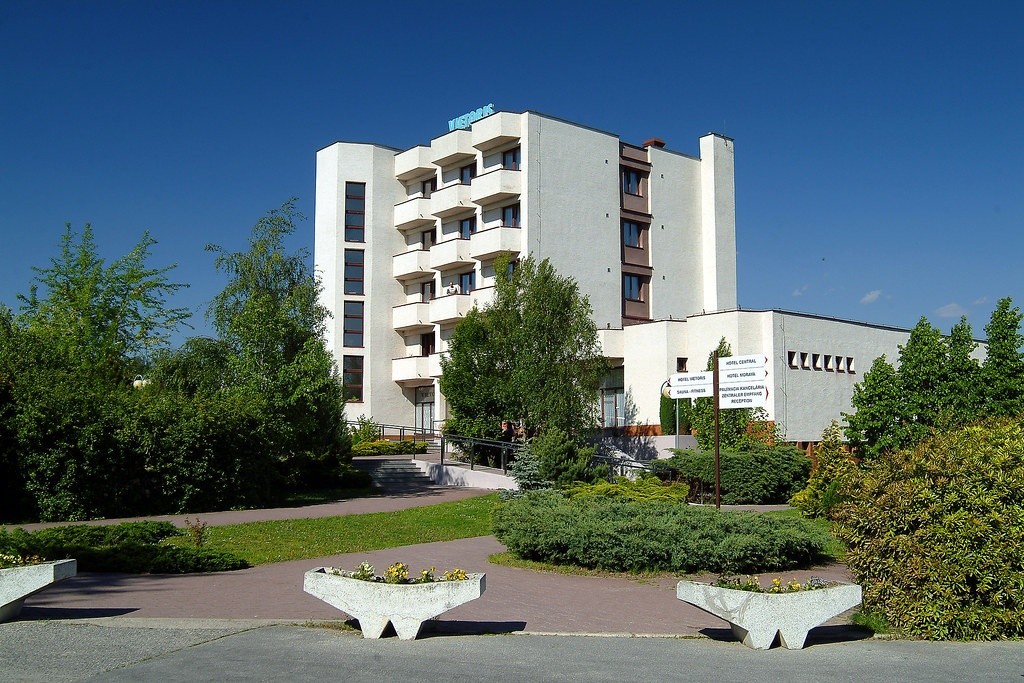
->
[329, 559, 470, 585]
[708, 568, 829, 594]
[0, 552, 46, 569]
